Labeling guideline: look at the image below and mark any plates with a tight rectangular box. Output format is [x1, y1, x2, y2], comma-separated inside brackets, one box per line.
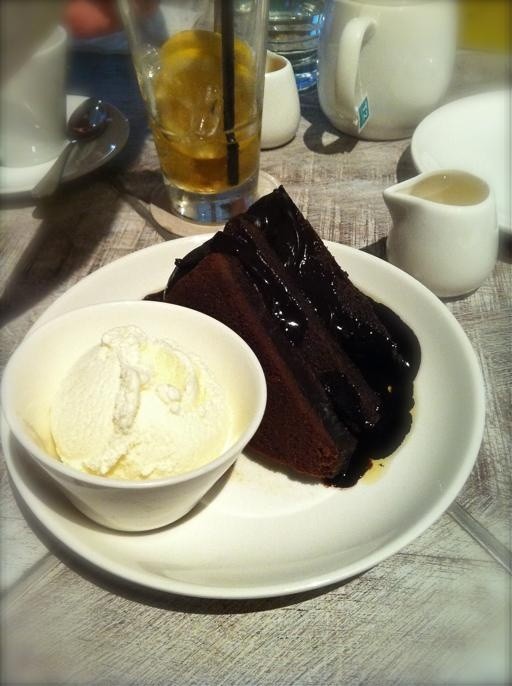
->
[0, 90, 130, 196]
[412, 89, 511, 233]
[0, 230, 488, 601]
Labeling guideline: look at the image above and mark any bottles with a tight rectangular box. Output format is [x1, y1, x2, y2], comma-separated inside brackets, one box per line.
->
[268, 2, 317, 88]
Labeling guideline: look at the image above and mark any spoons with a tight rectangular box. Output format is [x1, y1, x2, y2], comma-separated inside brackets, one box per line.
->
[31, 93, 108, 199]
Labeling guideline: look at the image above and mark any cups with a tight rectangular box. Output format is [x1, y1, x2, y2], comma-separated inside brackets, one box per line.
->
[121, 1, 269, 221]
[261, 49, 302, 150]
[384, 170, 501, 296]
[318, 2, 458, 143]
[1, 17, 67, 166]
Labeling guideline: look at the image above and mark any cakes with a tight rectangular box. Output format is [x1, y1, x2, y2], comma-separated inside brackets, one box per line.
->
[143, 183, 406, 479]
